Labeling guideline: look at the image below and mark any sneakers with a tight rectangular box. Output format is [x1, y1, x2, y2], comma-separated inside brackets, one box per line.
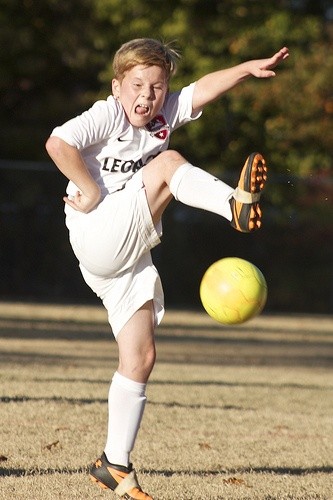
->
[228, 153, 266, 232]
[89, 452, 154, 500]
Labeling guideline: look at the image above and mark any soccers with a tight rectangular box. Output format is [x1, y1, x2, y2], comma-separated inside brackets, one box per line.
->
[200, 257, 268, 325]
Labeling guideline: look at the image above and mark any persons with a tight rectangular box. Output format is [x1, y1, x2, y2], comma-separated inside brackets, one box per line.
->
[44, 37, 289, 500]
